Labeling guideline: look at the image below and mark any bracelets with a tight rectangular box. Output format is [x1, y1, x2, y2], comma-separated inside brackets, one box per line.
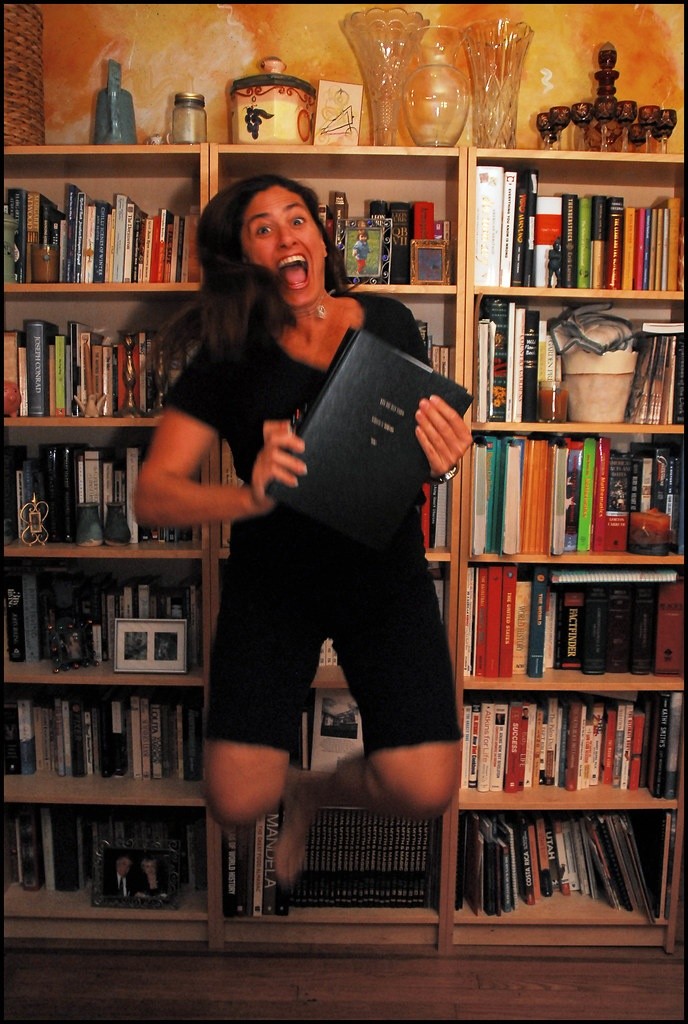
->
[433, 465, 456, 485]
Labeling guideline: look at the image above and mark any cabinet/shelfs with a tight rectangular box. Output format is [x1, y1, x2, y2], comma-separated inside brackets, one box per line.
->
[210, 142, 468, 958]
[445, 147, 684, 962]
[4, 143, 217, 952]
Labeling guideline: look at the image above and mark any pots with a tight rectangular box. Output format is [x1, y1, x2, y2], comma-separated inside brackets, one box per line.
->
[229, 57, 316, 145]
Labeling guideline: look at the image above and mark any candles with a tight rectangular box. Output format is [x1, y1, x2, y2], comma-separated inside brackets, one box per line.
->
[538, 381, 568, 423]
[630, 508, 670, 556]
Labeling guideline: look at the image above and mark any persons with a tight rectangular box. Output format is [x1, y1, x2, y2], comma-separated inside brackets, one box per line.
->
[134, 173, 474, 884]
[352, 229, 373, 275]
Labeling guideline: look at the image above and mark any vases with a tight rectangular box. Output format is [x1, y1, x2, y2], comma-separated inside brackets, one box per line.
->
[344, 6, 430, 146]
[561, 350, 638, 423]
[402, 25, 470, 147]
[460, 19, 534, 150]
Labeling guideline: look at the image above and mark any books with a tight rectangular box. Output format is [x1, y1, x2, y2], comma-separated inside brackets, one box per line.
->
[4, 161, 684, 919]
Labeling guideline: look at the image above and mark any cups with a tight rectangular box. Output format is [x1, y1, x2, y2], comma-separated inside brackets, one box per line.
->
[539, 381, 569, 424]
[30, 244, 59, 282]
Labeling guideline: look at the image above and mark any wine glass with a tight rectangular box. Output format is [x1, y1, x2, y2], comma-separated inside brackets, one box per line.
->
[629, 123, 646, 153]
[537, 111, 552, 150]
[616, 101, 637, 152]
[542, 131, 557, 150]
[550, 106, 571, 150]
[639, 105, 660, 153]
[570, 103, 593, 151]
[597, 100, 619, 151]
[659, 109, 677, 153]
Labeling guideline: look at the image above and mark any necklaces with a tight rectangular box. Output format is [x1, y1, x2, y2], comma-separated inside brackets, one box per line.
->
[318, 289, 336, 319]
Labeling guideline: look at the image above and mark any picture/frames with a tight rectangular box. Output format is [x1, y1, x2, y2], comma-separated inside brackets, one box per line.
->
[113, 618, 188, 674]
[91, 838, 181, 910]
[410, 239, 451, 286]
[335, 219, 392, 285]
[48, 620, 99, 674]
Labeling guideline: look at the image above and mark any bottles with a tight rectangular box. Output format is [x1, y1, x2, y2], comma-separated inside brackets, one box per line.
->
[172, 92, 207, 144]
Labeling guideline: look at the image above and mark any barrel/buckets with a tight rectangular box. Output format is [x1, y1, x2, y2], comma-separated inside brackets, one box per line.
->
[563, 349, 639, 423]
